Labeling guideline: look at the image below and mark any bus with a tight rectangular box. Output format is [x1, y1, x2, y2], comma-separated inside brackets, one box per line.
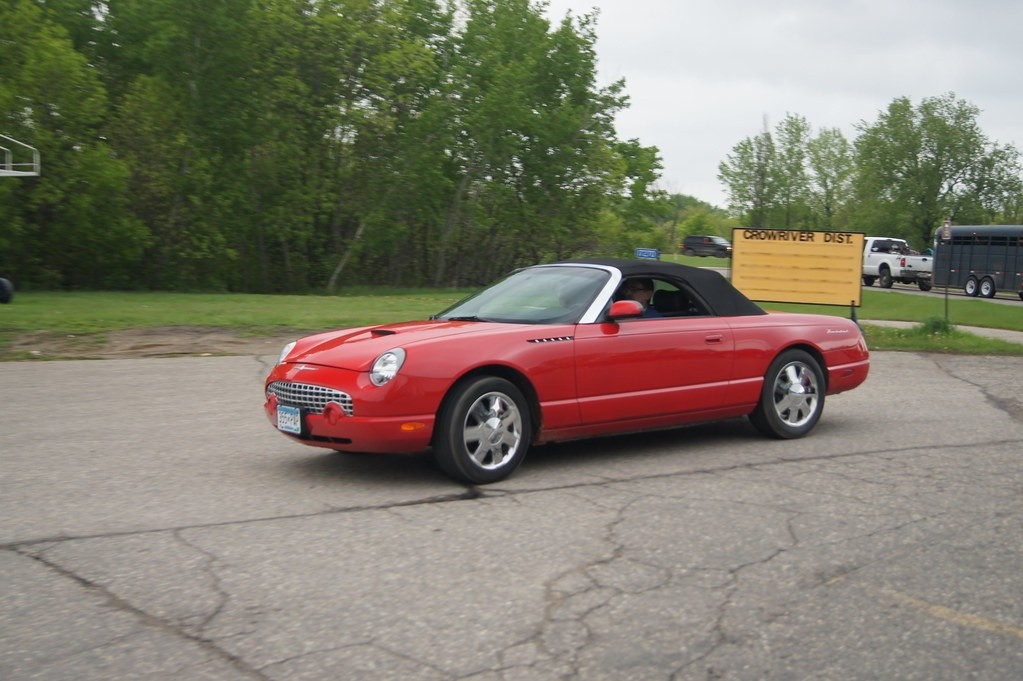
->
[931, 224, 1023, 299]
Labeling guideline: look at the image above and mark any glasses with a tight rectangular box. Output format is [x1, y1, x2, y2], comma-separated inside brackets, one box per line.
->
[622, 284, 649, 293]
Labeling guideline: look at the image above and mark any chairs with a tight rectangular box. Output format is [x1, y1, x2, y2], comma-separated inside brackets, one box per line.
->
[649, 289, 691, 317]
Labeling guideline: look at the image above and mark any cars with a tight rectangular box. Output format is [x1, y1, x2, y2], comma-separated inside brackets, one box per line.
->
[264, 257, 871, 486]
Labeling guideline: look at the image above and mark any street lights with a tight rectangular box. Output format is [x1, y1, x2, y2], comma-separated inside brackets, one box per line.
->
[940, 215, 952, 325]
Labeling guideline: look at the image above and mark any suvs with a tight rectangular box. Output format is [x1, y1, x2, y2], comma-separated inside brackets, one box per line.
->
[681, 234, 733, 258]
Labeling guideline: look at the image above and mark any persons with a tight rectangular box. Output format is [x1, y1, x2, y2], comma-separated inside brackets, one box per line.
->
[616, 278, 660, 319]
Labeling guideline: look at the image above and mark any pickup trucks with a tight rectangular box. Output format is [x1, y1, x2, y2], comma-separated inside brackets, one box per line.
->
[861, 236, 934, 292]
